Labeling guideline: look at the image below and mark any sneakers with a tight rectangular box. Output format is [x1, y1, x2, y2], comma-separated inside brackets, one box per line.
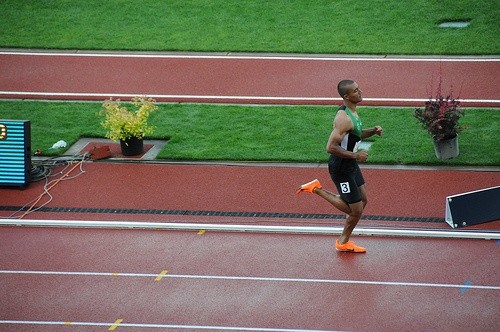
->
[334, 239, 367, 254]
[294, 178, 321, 196]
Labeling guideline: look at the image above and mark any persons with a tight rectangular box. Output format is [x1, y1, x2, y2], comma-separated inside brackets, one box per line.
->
[296, 80, 382, 253]
